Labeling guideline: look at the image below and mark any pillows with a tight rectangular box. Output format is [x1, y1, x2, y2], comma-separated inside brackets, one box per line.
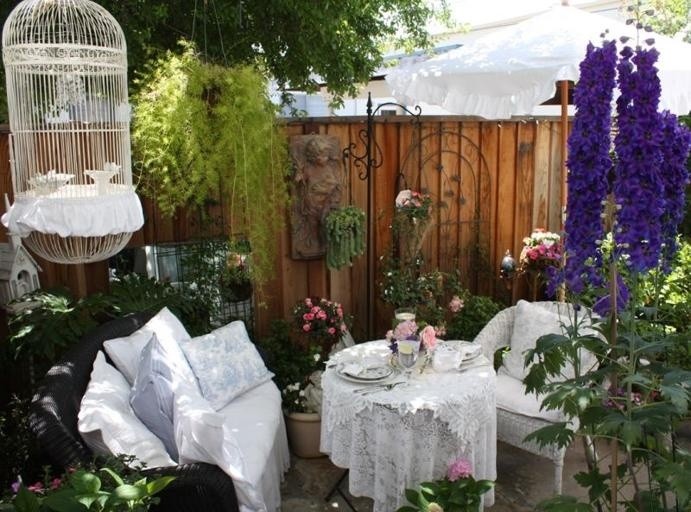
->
[74, 305, 275, 474]
[499, 300, 600, 394]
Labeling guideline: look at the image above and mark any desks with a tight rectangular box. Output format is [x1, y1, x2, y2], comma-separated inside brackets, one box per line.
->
[318, 338, 497, 511]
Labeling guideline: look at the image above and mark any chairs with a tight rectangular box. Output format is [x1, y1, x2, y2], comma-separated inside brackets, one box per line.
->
[466, 298, 619, 497]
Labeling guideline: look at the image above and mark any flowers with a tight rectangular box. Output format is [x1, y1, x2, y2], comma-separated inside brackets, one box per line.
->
[278, 295, 355, 416]
[397, 191, 435, 222]
[516, 228, 563, 277]
[383, 312, 439, 356]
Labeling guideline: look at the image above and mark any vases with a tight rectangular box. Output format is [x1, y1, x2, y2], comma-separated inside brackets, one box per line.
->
[286, 414, 328, 458]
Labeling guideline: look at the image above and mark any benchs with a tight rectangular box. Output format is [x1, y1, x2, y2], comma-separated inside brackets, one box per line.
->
[16, 300, 284, 511]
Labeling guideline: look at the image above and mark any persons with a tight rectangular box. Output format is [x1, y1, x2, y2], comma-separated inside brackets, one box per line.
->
[294, 138, 336, 250]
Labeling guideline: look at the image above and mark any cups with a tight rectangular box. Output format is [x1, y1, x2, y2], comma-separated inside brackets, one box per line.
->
[395, 308, 417, 322]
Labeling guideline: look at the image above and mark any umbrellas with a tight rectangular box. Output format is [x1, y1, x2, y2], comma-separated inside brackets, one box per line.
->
[383, 1, 691, 306]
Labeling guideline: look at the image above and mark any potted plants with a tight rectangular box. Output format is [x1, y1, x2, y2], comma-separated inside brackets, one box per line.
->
[216, 247, 274, 303]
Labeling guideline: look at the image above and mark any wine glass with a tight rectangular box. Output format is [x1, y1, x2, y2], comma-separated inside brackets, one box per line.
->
[397, 335, 422, 392]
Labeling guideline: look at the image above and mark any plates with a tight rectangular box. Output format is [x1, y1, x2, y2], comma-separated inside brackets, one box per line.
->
[427, 340, 483, 365]
[335, 356, 394, 384]
